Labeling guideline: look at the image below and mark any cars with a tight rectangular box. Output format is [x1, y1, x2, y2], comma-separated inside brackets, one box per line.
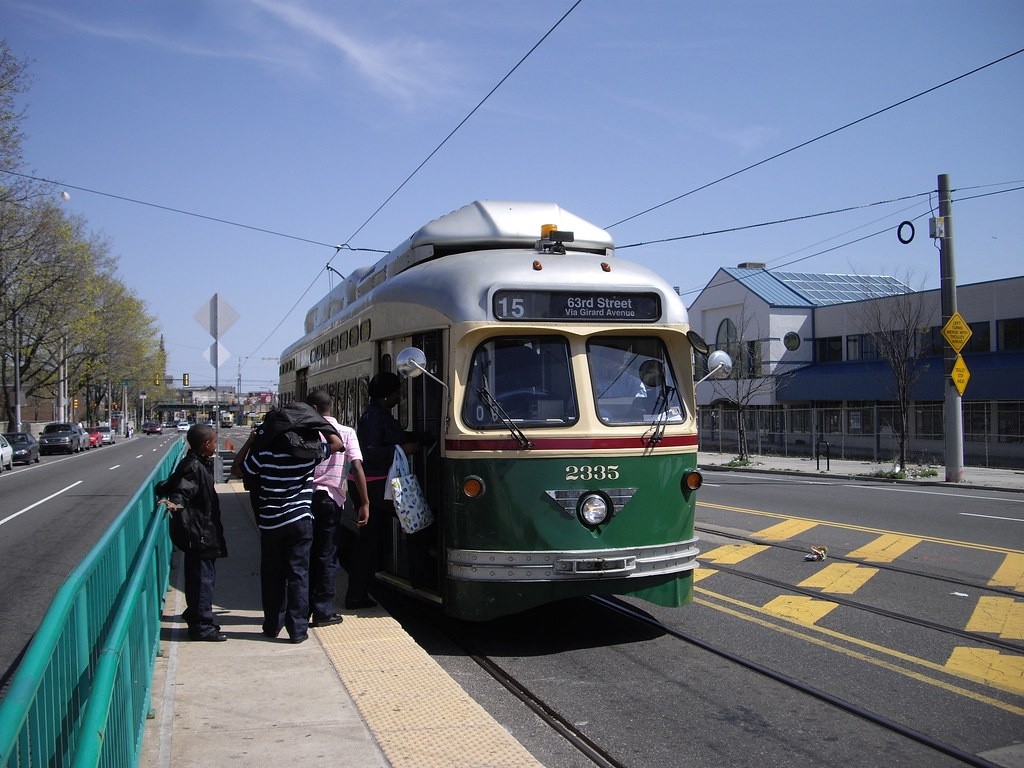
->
[0, 422, 117, 474]
[140, 420, 212, 436]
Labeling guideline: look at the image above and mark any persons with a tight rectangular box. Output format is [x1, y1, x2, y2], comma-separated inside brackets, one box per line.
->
[346, 372, 432, 611]
[154, 424, 229, 643]
[232, 388, 369, 644]
[125, 423, 134, 438]
[464, 341, 648, 424]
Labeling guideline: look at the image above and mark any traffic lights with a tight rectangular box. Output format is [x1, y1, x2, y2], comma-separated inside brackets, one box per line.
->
[73, 399, 79, 409]
[154, 372, 161, 386]
[183, 373, 191, 386]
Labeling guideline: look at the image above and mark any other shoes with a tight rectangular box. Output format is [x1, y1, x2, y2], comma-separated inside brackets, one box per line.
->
[200, 630, 227, 641]
[312, 614, 343, 626]
[348, 600, 378, 611]
[183, 613, 219, 630]
[292, 634, 309, 644]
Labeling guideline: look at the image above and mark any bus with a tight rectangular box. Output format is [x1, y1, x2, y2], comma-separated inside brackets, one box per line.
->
[219, 412, 234, 428]
[276, 198, 733, 626]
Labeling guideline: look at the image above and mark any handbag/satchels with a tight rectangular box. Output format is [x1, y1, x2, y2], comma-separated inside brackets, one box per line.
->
[394, 444, 434, 534]
[385, 449, 406, 501]
